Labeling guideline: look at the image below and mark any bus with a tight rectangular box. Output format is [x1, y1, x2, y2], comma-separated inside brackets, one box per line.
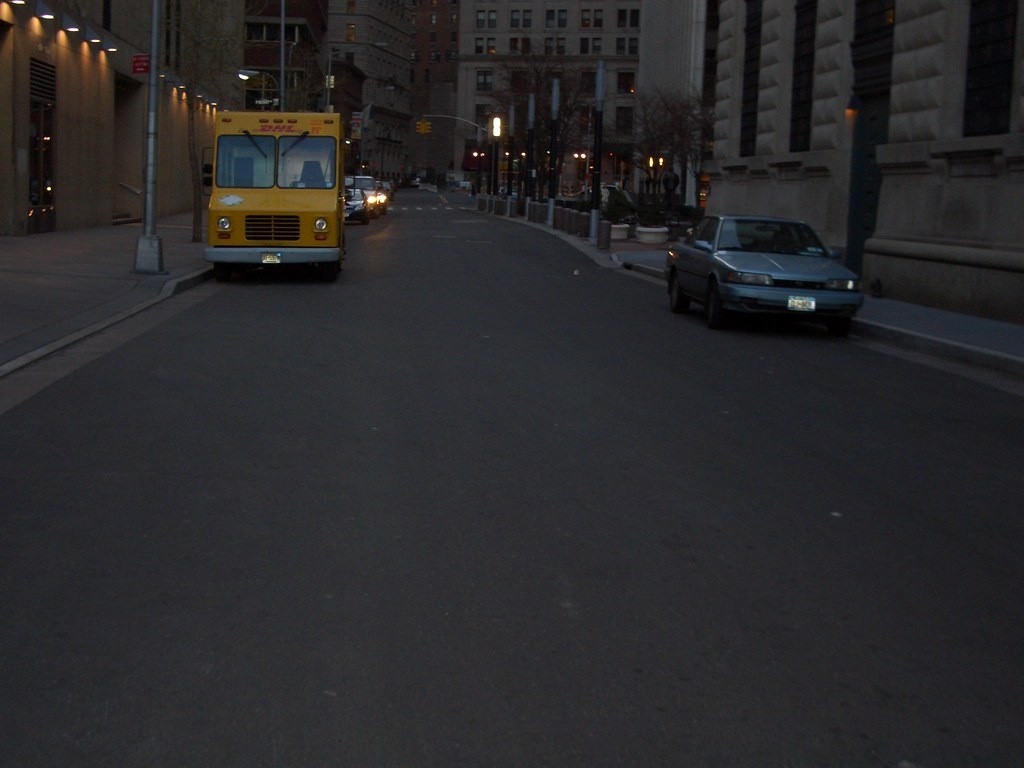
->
[201, 111, 360, 284]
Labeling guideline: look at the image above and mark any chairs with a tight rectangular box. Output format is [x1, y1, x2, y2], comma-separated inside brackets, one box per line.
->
[772, 229, 793, 247]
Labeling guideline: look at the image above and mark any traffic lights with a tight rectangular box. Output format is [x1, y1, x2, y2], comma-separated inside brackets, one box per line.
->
[416, 120, 431, 135]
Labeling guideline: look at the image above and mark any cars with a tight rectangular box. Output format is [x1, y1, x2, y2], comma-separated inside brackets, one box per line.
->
[343, 176, 420, 225]
[664, 214, 862, 341]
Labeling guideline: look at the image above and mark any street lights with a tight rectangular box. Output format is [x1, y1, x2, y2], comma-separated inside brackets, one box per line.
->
[325, 41, 388, 113]
[506, 104, 515, 217]
[590, 57, 605, 249]
[548, 77, 560, 226]
[360, 87, 395, 176]
[491, 115, 500, 215]
[525, 94, 535, 221]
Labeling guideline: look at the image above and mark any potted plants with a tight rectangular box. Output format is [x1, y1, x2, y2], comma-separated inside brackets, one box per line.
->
[634, 208, 670, 245]
[601, 186, 634, 240]
